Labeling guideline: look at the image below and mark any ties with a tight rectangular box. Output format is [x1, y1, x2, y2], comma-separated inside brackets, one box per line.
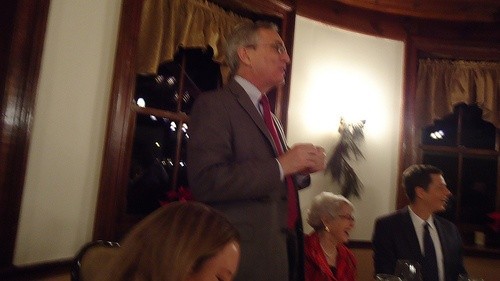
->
[423, 223, 439, 281]
[260, 94, 299, 230]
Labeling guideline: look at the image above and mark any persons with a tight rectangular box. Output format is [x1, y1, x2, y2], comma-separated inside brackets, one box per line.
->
[371, 164, 469, 281]
[187, 21, 328, 281]
[300, 192, 360, 281]
[90, 201, 239, 281]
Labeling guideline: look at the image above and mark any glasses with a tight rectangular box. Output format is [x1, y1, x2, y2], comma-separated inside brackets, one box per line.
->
[339, 214, 357, 223]
[247, 44, 288, 55]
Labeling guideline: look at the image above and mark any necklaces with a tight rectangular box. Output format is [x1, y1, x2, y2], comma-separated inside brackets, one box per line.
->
[319, 239, 338, 259]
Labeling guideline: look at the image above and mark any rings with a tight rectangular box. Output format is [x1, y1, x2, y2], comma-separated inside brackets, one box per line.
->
[322, 152, 326, 157]
